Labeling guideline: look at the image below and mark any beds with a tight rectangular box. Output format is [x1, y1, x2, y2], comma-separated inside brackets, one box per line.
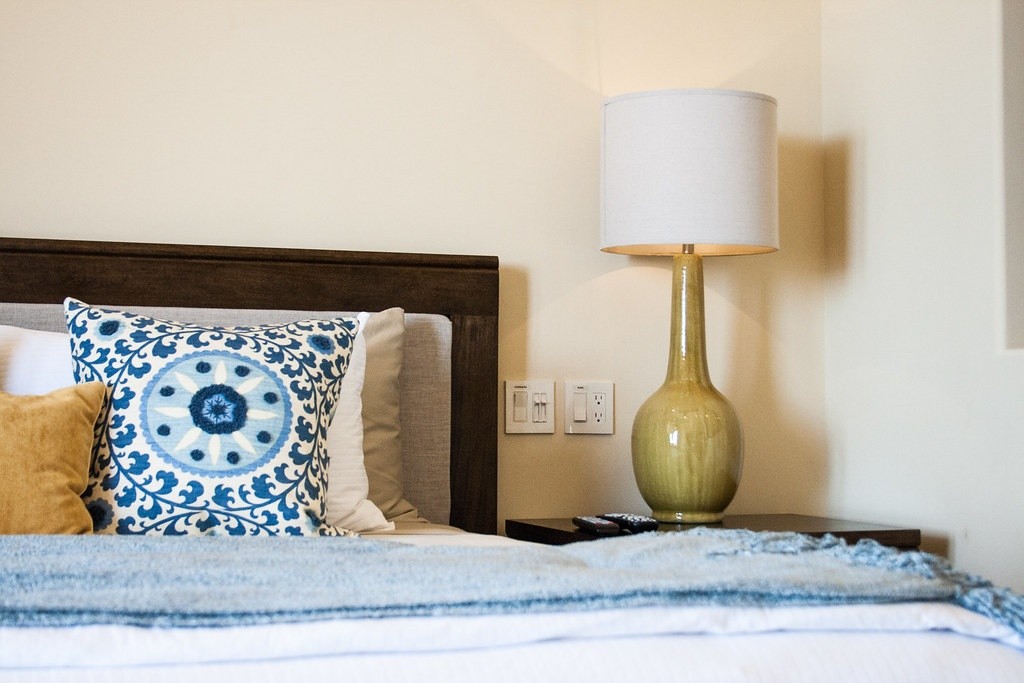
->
[0, 237, 1024, 683]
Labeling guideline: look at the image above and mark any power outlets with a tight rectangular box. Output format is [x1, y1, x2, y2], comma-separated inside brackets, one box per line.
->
[565, 381, 614, 435]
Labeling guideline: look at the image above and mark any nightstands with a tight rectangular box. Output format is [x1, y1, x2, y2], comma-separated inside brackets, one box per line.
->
[505, 514, 921, 554]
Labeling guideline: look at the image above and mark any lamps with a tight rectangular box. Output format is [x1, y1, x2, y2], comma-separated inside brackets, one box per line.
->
[601, 88, 779, 526]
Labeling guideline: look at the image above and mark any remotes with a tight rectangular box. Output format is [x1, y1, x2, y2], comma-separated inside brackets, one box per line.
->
[572, 513, 660, 533]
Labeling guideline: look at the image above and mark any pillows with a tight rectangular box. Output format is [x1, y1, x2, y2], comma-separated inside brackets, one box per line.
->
[0, 310, 397, 537]
[64, 297, 362, 537]
[362, 306, 430, 524]
[0, 381, 106, 535]
